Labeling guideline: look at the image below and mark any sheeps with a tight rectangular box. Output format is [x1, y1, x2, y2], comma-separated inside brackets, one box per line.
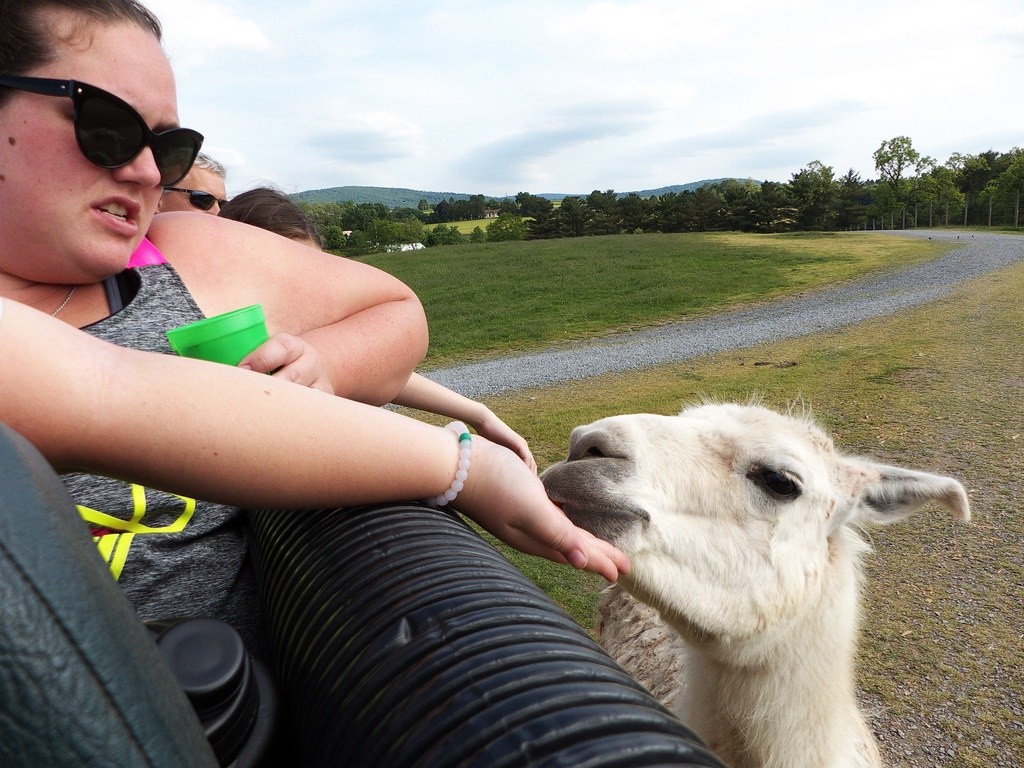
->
[538, 402, 971, 768]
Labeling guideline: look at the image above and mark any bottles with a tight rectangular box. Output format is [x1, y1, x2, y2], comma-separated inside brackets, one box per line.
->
[153, 620, 247, 720]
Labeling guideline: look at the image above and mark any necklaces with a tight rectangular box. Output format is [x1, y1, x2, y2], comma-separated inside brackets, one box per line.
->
[52, 285, 79, 316]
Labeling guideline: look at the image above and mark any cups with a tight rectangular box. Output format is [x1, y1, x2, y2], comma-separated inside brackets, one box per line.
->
[165, 305, 273, 376]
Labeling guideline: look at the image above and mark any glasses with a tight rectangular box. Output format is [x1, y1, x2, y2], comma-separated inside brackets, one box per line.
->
[0, 76, 204, 188]
[162, 186, 228, 211]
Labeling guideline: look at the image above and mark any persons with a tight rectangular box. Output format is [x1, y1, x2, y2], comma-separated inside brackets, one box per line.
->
[0, 0, 628, 635]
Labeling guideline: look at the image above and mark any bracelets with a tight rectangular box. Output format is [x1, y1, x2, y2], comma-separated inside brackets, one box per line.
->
[430, 421, 471, 506]
